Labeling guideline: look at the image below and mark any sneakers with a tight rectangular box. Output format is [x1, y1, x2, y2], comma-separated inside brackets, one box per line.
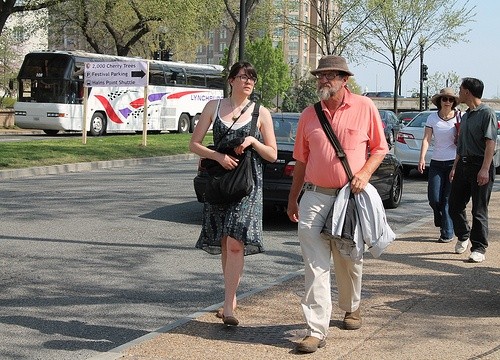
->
[343, 307, 362, 329]
[297, 336, 326, 352]
[468, 251, 485, 263]
[455, 237, 469, 254]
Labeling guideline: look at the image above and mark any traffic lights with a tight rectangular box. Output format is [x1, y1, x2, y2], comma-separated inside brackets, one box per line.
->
[154, 51, 160, 60]
[423, 64, 428, 81]
[161, 50, 173, 61]
[426, 96, 430, 110]
[421, 99, 426, 110]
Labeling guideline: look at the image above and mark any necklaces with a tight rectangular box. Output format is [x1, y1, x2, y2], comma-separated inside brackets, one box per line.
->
[229, 97, 247, 120]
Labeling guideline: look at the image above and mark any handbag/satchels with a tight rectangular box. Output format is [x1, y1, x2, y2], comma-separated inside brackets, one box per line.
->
[194, 148, 257, 205]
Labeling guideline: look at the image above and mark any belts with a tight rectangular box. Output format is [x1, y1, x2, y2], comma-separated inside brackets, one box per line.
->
[303, 182, 341, 197]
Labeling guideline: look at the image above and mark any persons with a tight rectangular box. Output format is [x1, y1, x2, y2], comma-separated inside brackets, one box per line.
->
[189, 62, 277, 325]
[448, 78, 498, 262]
[286, 56, 390, 353]
[418, 87, 466, 243]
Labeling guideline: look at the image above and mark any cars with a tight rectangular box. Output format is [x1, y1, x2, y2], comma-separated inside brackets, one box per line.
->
[363, 91, 430, 98]
[396, 111, 422, 126]
[375, 110, 408, 146]
[261, 114, 405, 214]
[393, 110, 500, 174]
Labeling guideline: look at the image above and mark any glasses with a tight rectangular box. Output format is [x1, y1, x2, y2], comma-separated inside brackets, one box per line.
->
[234, 74, 257, 84]
[443, 97, 454, 103]
[315, 71, 340, 80]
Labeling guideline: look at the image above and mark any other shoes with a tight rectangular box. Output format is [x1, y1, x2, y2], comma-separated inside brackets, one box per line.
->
[217, 306, 224, 318]
[439, 237, 454, 243]
[223, 316, 239, 325]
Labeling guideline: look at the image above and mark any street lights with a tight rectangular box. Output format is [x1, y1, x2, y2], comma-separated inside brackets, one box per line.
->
[417, 33, 425, 110]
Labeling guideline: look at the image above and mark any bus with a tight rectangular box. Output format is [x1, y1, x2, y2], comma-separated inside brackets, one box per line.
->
[9, 49, 226, 137]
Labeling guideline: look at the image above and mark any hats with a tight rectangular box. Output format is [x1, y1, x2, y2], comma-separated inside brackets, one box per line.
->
[310, 55, 354, 76]
[432, 87, 460, 107]
